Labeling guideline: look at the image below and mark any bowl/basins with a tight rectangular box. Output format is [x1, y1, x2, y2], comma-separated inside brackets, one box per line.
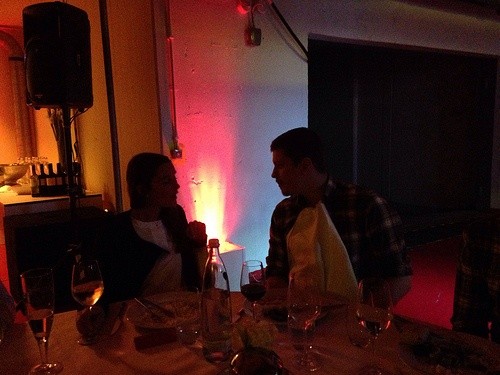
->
[126, 291, 197, 335]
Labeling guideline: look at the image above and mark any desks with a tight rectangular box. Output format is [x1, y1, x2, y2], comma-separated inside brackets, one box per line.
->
[0, 184, 103, 315]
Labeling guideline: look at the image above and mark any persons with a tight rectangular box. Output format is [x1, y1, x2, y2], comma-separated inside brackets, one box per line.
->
[265, 126, 412, 310]
[98, 153, 210, 306]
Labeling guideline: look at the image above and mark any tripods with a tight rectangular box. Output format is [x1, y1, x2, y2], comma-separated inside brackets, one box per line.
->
[50, 107, 85, 274]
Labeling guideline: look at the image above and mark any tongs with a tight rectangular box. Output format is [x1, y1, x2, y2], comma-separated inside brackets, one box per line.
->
[135, 296, 176, 324]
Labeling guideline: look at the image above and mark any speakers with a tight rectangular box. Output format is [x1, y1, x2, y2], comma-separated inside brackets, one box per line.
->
[22, 1, 93, 113]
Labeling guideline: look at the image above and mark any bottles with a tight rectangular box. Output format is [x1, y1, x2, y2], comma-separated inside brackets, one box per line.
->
[217, 345, 295, 375]
[15, 155, 82, 198]
[200, 239, 232, 365]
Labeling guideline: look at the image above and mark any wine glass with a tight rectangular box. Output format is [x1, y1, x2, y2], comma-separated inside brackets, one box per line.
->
[239, 260, 266, 322]
[346, 299, 372, 375]
[355, 276, 394, 375]
[20, 267, 64, 375]
[287, 272, 322, 372]
[71, 259, 106, 345]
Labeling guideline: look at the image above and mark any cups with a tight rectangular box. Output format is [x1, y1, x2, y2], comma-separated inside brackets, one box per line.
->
[175, 286, 202, 346]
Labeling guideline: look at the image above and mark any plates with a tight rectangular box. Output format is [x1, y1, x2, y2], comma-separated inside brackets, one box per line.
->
[398, 328, 500, 375]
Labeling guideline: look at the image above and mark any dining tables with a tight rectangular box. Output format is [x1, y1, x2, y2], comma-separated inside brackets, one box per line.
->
[0, 288, 500, 375]
